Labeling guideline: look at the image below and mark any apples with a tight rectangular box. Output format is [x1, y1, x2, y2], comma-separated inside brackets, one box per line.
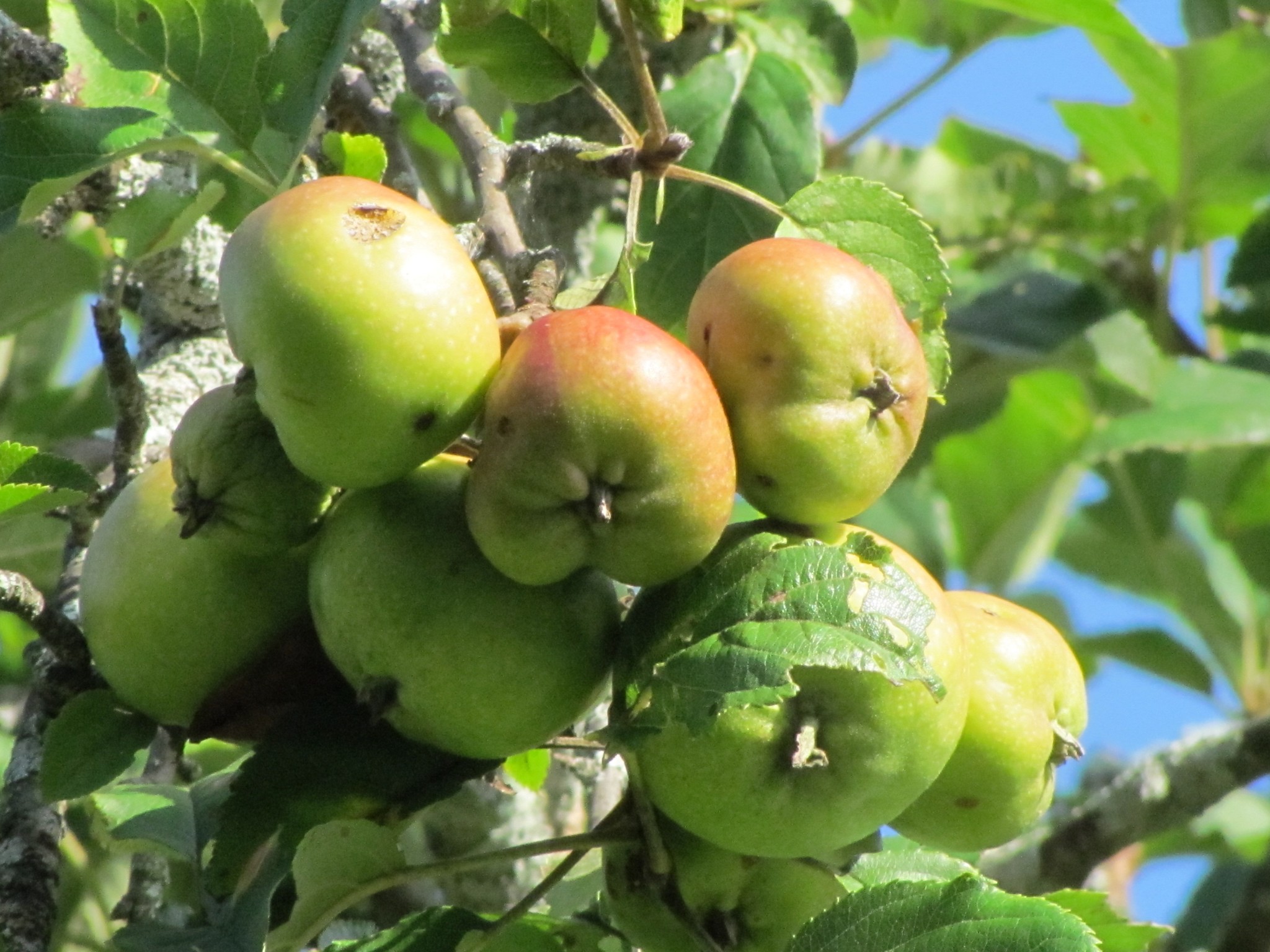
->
[75, 175, 1085, 952]
